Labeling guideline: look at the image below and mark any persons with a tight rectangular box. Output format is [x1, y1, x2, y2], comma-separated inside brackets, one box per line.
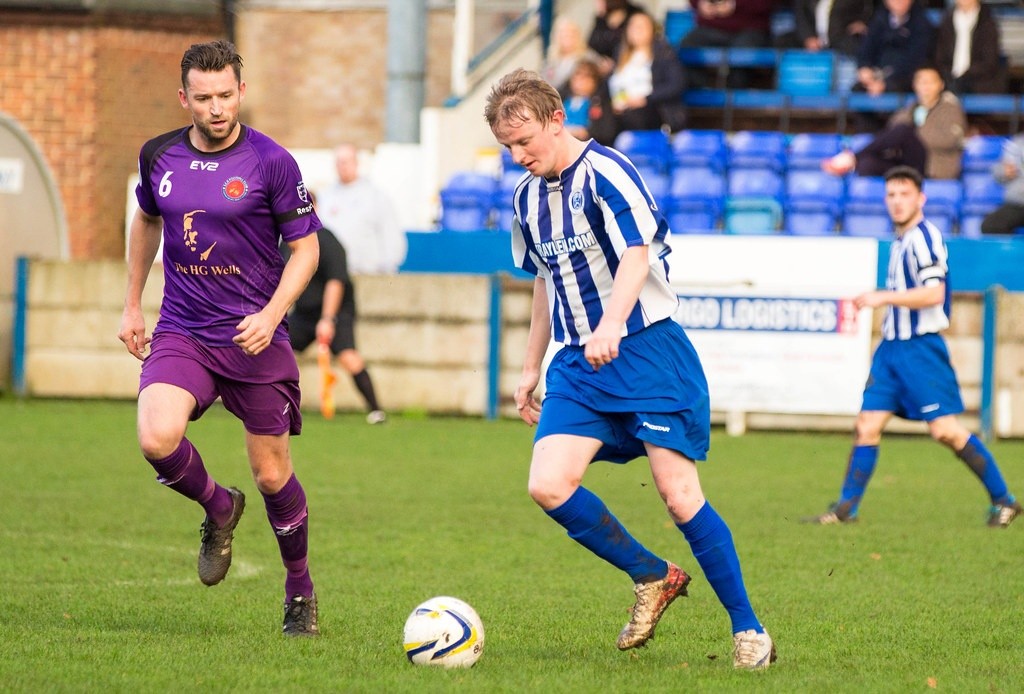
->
[546, 0, 702, 148]
[820, 65, 965, 181]
[119, 42, 320, 635]
[798, 167, 1023, 527]
[318, 145, 409, 277]
[793, 1, 1002, 94]
[484, 69, 777, 673]
[279, 193, 387, 423]
[981, 131, 1024, 234]
[681, 0, 777, 90]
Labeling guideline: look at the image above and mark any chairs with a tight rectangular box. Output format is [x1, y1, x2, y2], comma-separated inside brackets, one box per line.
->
[433, 13, 1011, 234]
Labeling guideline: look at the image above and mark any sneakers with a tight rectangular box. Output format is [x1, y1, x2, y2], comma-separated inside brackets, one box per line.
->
[197, 487, 245, 585]
[989, 501, 1022, 528]
[617, 559, 692, 650]
[732, 624, 777, 673]
[817, 502, 845, 526]
[281, 592, 319, 636]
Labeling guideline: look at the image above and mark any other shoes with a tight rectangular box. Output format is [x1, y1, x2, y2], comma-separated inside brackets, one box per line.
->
[366, 409, 386, 425]
[824, 148, 860, 177]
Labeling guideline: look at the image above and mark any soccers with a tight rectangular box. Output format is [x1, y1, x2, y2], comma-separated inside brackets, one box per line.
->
[403, 596, 487, 670]
